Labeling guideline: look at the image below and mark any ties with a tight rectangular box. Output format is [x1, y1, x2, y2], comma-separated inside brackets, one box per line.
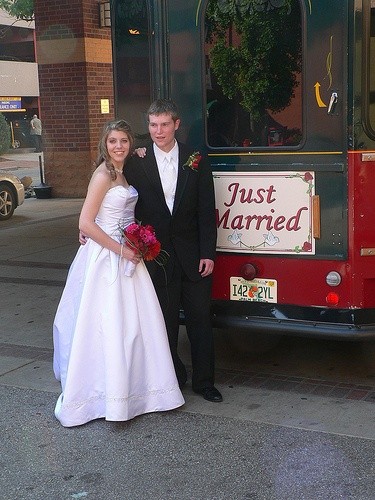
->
[162, 154, 176, 216]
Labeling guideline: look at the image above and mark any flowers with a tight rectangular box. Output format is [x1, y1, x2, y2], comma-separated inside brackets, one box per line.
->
[95, 215, 170, 289]
[182, 151, 202, 173]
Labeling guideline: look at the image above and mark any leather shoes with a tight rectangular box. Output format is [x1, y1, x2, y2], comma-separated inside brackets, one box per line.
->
[192, 383, 224, 402]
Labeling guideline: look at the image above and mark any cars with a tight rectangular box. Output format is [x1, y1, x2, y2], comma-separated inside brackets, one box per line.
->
[9, 126, 33, 149]
[0, 170, 25, 221]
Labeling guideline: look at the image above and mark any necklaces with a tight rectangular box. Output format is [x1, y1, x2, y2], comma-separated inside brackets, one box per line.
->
[115, 168, 124, 174]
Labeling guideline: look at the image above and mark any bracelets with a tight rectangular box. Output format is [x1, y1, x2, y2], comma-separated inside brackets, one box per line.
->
[121, 245, 124, 257]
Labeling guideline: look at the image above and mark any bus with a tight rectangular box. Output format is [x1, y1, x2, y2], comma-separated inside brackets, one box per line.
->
[109, 0, 375, 345]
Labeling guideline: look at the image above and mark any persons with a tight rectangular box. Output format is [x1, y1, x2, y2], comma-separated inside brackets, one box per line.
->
[78, 99, 222, 402]
[30, 114, 42, 152]
[53, 120, 185, 427]
